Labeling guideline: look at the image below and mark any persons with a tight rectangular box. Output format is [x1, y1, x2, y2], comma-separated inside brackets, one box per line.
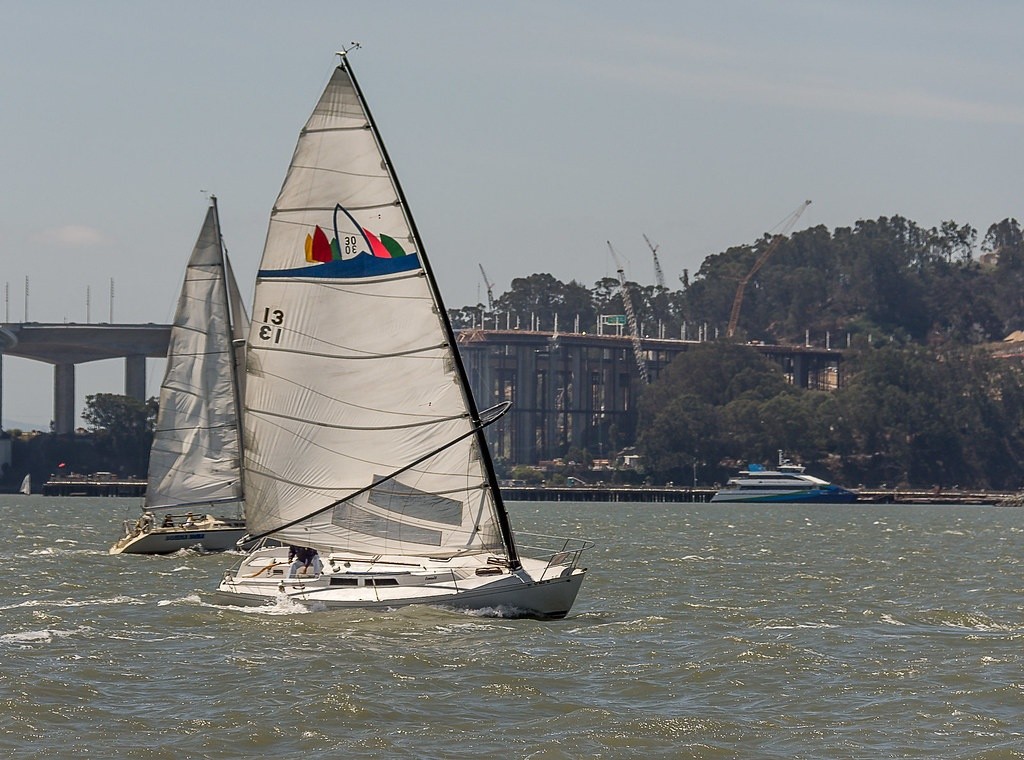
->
[287, 545, 320, 578]
[179, 511, 194, 529]
[161, 514, 174, 528]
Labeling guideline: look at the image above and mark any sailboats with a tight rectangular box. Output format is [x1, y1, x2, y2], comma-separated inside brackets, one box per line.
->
[110, 198, 251, 554]
[213, 44, 594, 622]
[20, 474, 31, 496]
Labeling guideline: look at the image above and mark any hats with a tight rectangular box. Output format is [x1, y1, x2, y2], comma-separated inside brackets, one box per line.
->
[185, 512, 192, 515]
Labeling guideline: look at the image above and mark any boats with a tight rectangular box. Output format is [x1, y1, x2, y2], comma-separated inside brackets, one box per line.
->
[710, 449, 858, 503]
